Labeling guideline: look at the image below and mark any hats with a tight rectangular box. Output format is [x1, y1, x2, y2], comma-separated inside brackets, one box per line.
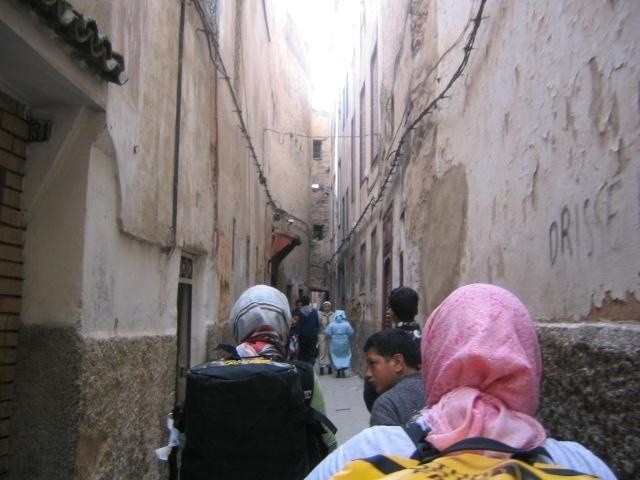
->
[389, 286, 419, 322]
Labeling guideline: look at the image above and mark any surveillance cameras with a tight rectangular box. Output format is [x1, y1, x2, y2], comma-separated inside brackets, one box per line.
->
[30, 119, 53, 143]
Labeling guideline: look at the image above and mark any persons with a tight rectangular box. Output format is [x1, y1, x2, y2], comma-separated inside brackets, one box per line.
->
[288, 296, 355, 377]
[181, 284, 336, 480]
[303, 283, 619, 480]
[363, 286, 427, 427]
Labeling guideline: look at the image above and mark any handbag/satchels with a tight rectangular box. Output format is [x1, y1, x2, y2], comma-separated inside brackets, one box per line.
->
[308, 336, 320, 357]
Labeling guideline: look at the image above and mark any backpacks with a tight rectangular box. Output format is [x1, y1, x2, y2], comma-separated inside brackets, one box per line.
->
[179, 355, 314, 480]
[328, 421, 604, 480]
